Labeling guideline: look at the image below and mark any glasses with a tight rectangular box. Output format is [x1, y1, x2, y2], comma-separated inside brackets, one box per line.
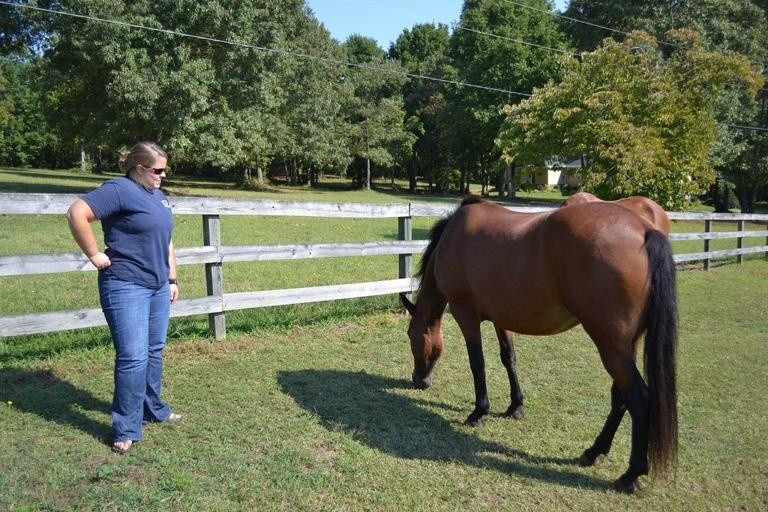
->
[145, 166, 165, 174]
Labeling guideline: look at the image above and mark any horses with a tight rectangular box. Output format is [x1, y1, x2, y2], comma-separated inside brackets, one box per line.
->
[398, 193, 680, 500]
[556, 192, 672, 238]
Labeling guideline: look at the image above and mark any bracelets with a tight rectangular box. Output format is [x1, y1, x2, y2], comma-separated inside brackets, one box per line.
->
[167, 278, 177, 285]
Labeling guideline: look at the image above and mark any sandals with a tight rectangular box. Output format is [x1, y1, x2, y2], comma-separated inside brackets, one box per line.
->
[112, 440, 133, 452]
[142, 412, 182, 424]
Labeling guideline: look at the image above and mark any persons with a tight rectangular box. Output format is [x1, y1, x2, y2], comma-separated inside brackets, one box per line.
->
[63, 141, 183, 454]
